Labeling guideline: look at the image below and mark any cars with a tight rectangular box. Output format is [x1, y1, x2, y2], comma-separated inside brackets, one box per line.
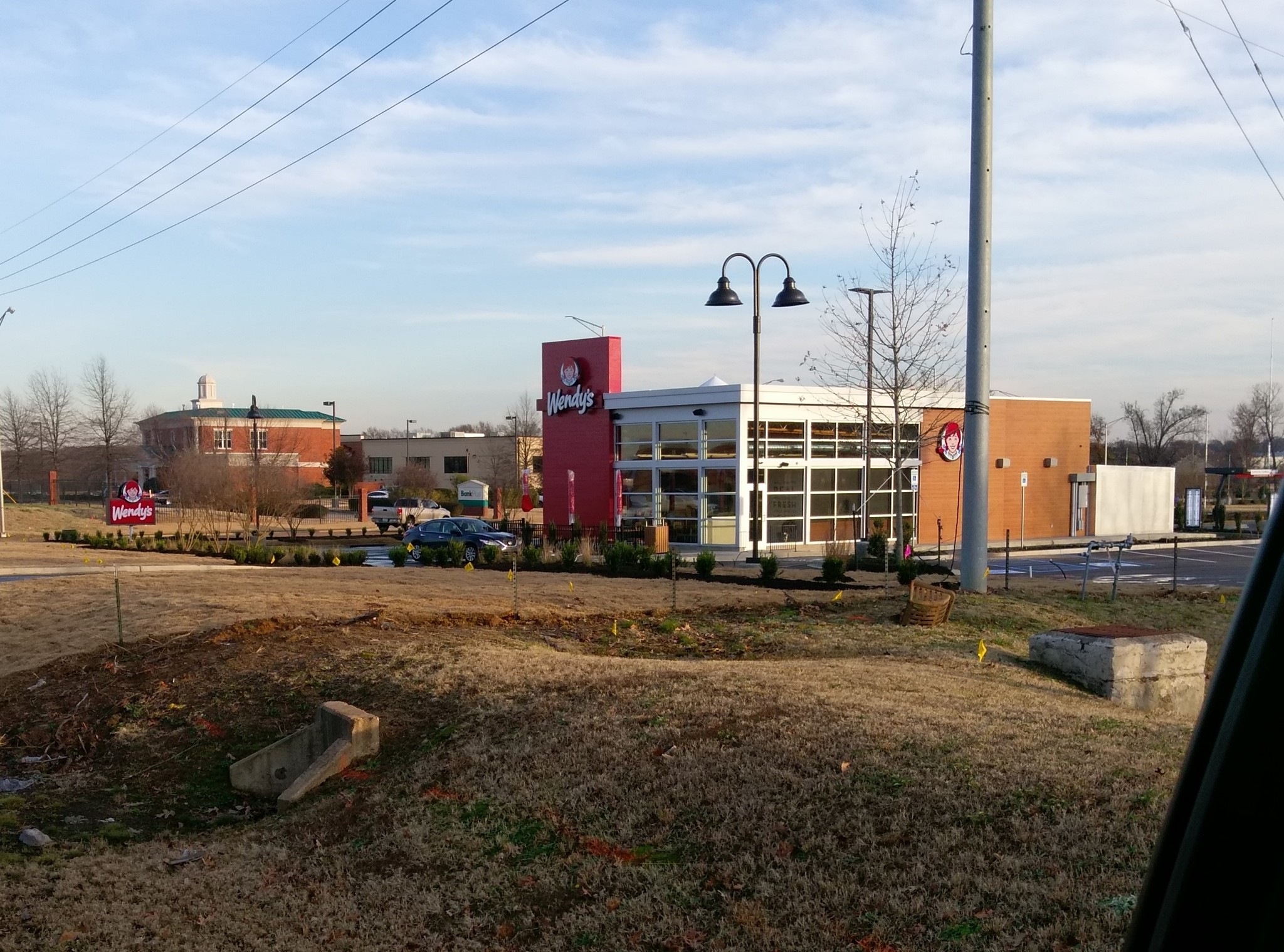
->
[102, 483, 171, 506]
[402, 517, 520, 566]
[367, 486, 398, 498]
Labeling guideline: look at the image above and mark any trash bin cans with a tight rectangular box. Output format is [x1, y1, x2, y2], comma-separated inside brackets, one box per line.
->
[644, 517, 669, 554]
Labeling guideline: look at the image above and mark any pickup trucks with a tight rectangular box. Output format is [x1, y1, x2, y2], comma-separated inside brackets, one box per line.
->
[371, 497, 452, 533]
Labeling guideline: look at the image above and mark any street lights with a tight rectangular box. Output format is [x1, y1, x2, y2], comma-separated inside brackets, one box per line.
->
[849, 287, 892, 542]
[244, 394, 266, 531]
[407, 419, 417, 466]
[31, 421, 44, 503]
[1105, 416, 1132, 466]
[323, 401, 336, 496]
[505, 416, 520, 489]
[704, 253, 810, 565]
